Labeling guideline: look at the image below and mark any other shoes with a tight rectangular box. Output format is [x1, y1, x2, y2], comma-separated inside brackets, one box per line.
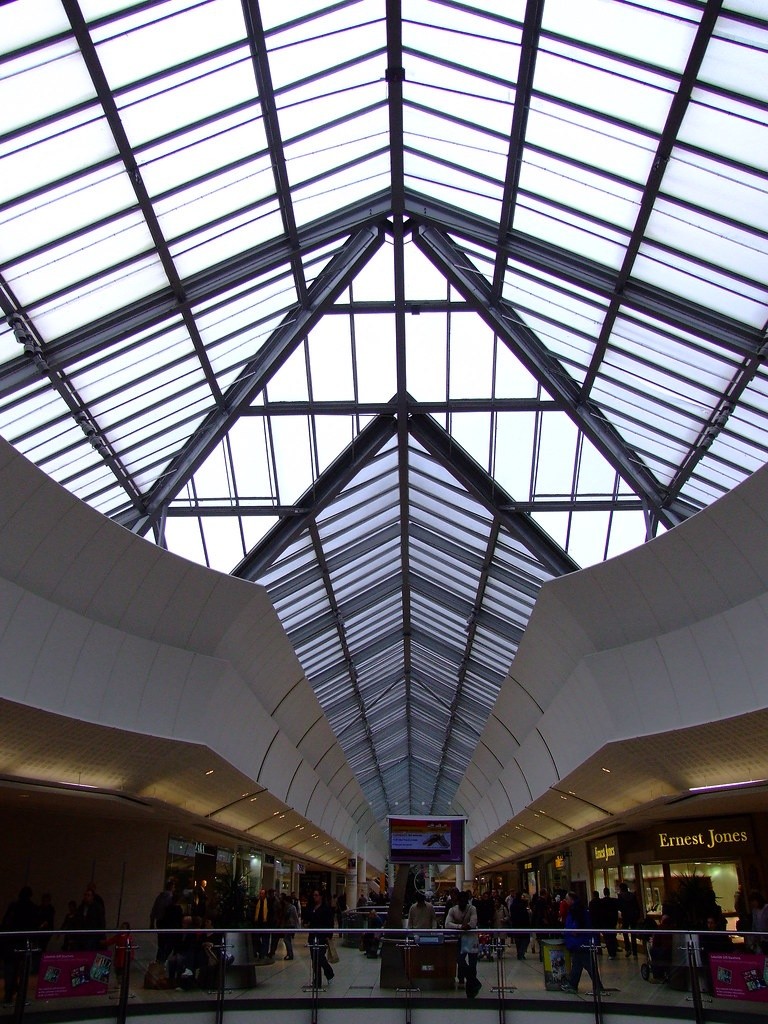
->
[327, 976, 334, 985]
[284, 954, 294, 960]
[561, 984, 578, 993]
[467, 985, 481, 998]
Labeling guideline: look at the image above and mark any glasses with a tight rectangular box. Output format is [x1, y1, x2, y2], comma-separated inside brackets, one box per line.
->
[313, 894, 320, 897]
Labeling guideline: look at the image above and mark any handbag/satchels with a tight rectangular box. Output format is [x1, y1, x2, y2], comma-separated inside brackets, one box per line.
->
[460, 933, 479, 954]
[218, 945, 235, 966]
[326, 938, 339, 964]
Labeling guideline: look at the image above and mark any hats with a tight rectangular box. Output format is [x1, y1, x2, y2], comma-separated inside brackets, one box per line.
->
[268, 888, 276, 895]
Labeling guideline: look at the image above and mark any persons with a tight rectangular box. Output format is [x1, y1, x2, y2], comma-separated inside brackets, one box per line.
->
[442, 887, 510, 1001]
[0, 887, 55, 1008]
[651, 884, 768, 994]
[407, 890, 436, 937]
[60, 883, 106, 951]
[324, 891, 346, 939]
[303, 888, 335, 988]
[616, 884, 641, 959]
[244, 888, 301, 960]
[104, 922, 136, 989]
[560, 892, 606, 996]
[357, 892, 388, 955]
[148, 878, 225, 989]
[588, 882, 619, 960]
[504, 888, 579, 960]
